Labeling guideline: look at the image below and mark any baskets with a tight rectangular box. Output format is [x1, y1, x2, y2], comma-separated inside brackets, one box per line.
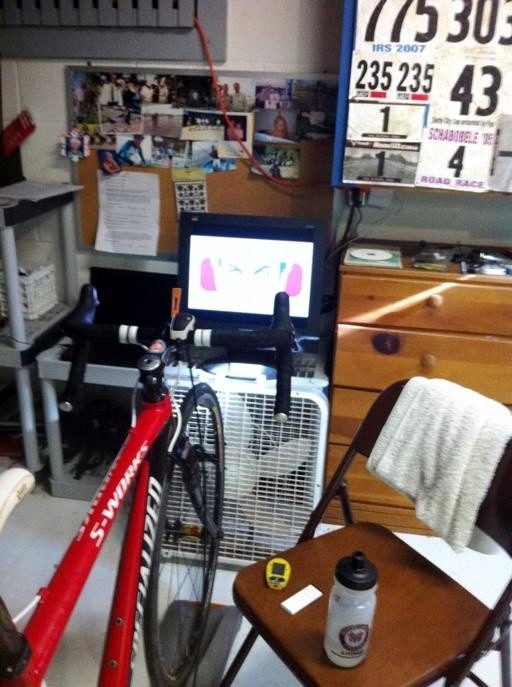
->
[0, 265, 59, 320]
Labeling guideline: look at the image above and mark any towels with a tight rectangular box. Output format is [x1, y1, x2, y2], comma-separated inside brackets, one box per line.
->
[366, 376, 512, 553]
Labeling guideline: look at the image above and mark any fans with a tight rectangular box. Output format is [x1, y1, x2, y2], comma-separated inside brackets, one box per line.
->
[160, 377, 330, 568]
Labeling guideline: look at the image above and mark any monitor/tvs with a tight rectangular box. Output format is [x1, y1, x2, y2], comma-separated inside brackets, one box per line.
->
[177, 209, 326, 337]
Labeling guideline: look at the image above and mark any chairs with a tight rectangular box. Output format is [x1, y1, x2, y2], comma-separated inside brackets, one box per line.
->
[219, 379, 512, 687]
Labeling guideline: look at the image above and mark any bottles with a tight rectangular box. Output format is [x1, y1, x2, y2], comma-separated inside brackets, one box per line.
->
[324, 549, 377, 667]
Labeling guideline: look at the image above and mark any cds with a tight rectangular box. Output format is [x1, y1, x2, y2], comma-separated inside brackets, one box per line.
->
[349, 248, 393, 261]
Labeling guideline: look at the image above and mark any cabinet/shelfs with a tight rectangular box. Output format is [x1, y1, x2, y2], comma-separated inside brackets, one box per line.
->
[1, 178, 84, 487]
[320, 239, 512, 537]
[36, 336, 330, 507]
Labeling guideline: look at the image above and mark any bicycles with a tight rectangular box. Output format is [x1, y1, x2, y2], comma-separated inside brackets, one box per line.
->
[0, 275, 296, 687]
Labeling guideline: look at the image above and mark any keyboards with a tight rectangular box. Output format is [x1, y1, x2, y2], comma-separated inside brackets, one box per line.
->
[169, 342, 328, 379]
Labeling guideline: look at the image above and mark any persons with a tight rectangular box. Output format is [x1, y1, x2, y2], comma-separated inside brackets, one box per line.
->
[56, 70, 336, 183]
